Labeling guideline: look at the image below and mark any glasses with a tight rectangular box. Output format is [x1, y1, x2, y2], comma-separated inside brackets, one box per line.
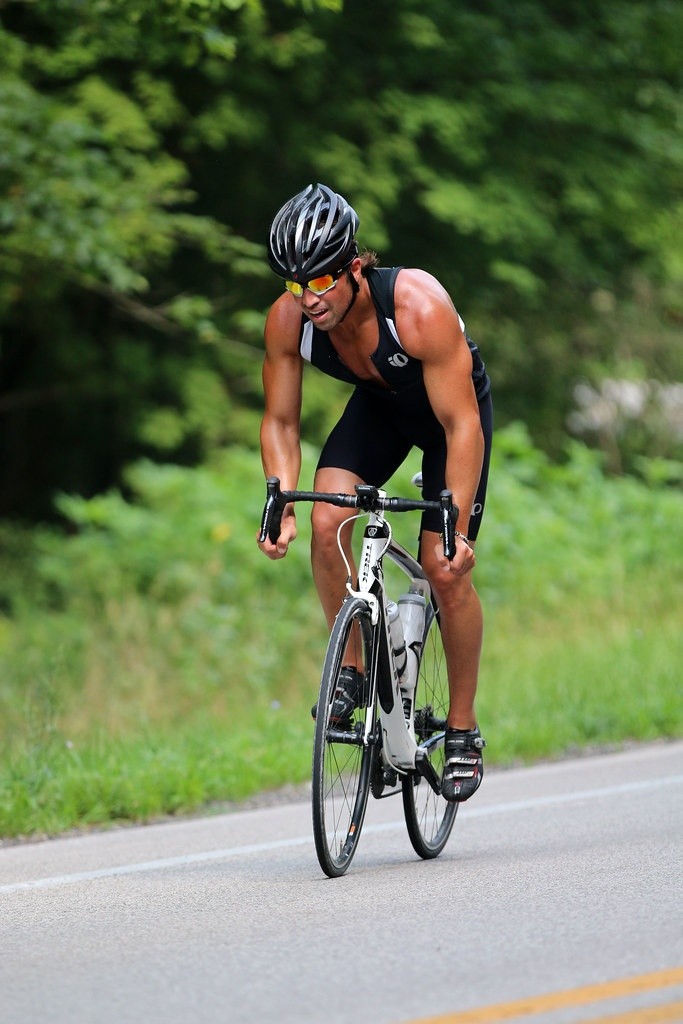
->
[283, 253, 357, 297]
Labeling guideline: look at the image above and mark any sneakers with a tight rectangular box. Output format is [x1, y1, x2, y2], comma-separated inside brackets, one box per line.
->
[440, 719, 486, 803]
[311, 664, 372, 724]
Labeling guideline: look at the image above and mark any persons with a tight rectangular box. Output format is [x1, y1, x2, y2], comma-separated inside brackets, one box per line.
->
[255, 181, 493, 801]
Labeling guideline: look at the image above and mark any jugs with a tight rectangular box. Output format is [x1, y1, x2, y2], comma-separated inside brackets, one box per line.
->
[386, 600, 408, 686]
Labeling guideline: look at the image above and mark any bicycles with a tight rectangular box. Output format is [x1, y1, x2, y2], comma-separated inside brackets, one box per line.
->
[260, 473, 468, 880]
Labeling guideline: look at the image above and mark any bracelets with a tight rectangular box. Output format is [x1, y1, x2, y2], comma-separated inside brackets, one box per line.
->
[455, 532, 468, 543]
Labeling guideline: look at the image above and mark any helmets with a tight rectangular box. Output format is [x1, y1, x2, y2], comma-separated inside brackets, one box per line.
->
[266, 181, 360, 284]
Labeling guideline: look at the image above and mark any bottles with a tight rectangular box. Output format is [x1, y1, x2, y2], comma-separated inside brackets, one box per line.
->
[397, 593, 425, 687]
